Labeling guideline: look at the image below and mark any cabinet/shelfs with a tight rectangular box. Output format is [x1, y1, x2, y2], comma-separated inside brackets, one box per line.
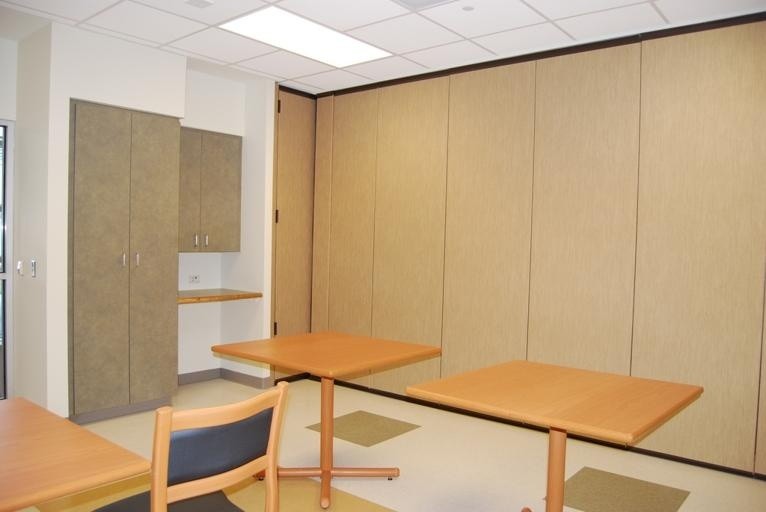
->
[182, 126, 244, 251]
[69, 96, 181, 426]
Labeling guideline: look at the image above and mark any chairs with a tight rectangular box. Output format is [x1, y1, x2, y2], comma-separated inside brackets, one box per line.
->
[150, 381, 286, 512]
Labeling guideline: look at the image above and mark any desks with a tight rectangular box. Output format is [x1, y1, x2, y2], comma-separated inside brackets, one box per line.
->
[1, 395, 150, 512]
